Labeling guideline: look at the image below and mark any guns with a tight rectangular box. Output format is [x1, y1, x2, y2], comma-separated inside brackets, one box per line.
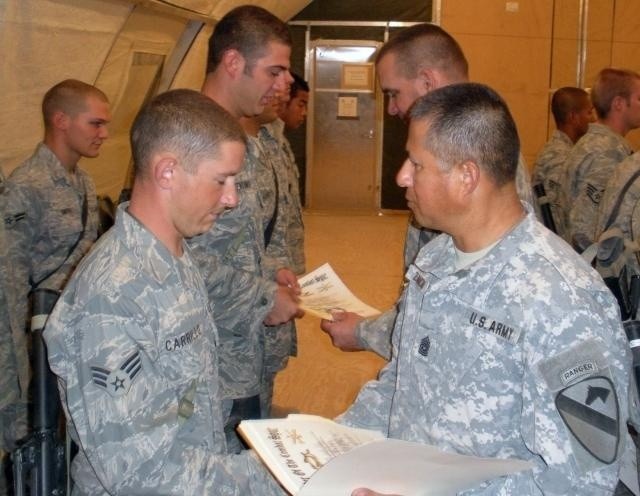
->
[532, 182, 639, 395]
[13, 288, 62, 496]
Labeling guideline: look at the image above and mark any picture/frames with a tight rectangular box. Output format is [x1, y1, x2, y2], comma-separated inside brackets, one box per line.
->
[341, 62, 374, 91]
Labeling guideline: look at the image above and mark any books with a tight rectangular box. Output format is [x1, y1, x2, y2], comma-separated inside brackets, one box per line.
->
[234, 407, 535, 496]
[290, 259, 385, 325]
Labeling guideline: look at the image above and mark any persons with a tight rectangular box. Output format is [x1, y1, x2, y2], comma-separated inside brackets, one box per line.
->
[557, 69, 640, 253]
[0, 78, 111, 495]
[186, 7, 303, 423]
[36, 86, 292, 495]
[587, 152, 640, 406]
[281, 71, 310, 363]
[327, 82, 640, 495]
[320, 25, 536, 364]
[530, 86, 599, 225]
[240, 87, 290, 416]
